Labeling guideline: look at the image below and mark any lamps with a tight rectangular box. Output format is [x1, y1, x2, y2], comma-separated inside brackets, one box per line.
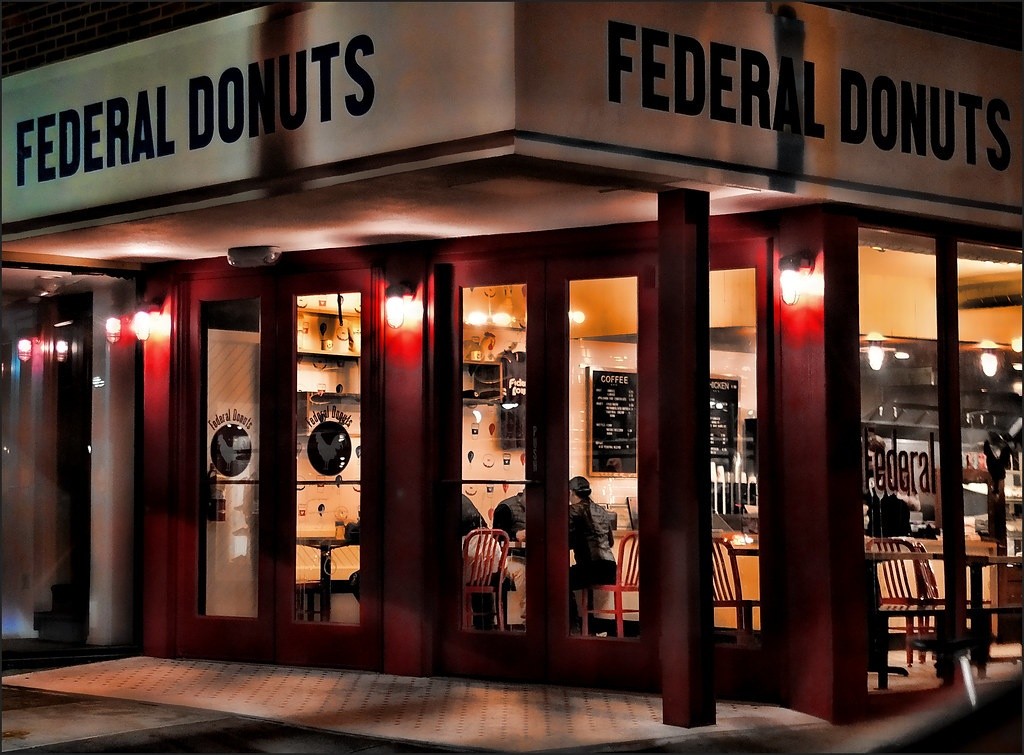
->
[865, 332, 886, 371]
[17, 326, 41, 362]
[462, 291, 492, 326]
[492, 288, 516, 325]
[974, 340, 1002, 378]
[132, 299, 163, 341]
[778, 248, 816, 306]
[380, 279, 417, 328]
[105, 312, 150, 342]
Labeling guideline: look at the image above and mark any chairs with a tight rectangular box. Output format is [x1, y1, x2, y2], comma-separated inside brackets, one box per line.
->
[580, 531, 641, 640]
[712, 536, 761, 648]
[461, 527, 510, 634]
[866, 540, 992, 669]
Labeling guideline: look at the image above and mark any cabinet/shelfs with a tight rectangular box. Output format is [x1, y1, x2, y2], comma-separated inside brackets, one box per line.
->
[296, 305, 361, 404]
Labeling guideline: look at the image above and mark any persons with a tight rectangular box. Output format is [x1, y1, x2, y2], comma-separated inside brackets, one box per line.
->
[346, 519, 361, 602]
[491, 488, 526, 633]
[565, 476, 616, 636]
[462, 494, 492, 630]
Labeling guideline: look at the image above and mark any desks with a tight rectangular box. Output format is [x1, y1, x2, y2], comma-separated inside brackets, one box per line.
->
[865, 551, 1023, 669]
[295, 537, 350, 624]
